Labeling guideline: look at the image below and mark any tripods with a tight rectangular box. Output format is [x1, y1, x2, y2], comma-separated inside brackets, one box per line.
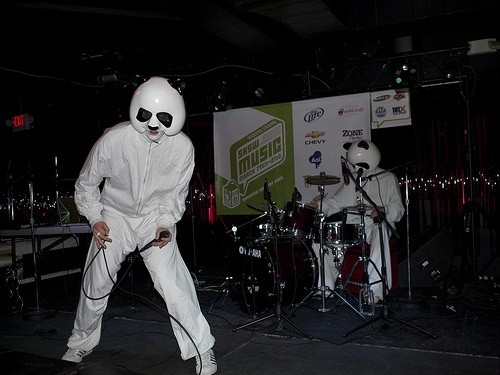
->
[232, 169, 437, 339]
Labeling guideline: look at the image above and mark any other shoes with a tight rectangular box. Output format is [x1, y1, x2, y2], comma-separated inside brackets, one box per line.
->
[313, 289, 334, 297]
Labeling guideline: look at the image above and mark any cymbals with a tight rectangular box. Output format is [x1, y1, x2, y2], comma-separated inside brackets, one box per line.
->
[246, 201, 283, 217]
[305, 175, 341, 186]
[343, 204, 377, 211]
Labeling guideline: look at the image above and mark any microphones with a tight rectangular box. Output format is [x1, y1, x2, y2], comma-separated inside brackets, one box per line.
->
[140, 231, 169, 253]
[294, 187, 302, 202]
[264, 179, 269, 200]
[341, 156, 350, 185]
[355, 168, 363, 191]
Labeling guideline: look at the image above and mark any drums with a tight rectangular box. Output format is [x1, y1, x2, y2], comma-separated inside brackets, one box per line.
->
[314, 221, 365, 247]
[277, 200, 317, 236]
[229, 234, 318, 316]
[340, 241, 392, 298]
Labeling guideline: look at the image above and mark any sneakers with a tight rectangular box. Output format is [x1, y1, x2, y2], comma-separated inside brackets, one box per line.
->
[61, 349, 94, 363]
[195, 349, 217, 375]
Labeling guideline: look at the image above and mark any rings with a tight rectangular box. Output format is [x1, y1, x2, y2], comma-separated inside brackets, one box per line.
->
[97, 232, 100, 236]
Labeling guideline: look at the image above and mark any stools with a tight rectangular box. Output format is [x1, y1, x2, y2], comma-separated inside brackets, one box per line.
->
[341, 238, 397, 298]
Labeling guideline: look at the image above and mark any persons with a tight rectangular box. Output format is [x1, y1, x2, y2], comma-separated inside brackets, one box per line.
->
[310, 139, 405, 304]
[61, 73, 217, 375]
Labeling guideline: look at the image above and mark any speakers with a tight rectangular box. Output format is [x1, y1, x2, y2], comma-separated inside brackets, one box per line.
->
[397, 228, 456, 289]
